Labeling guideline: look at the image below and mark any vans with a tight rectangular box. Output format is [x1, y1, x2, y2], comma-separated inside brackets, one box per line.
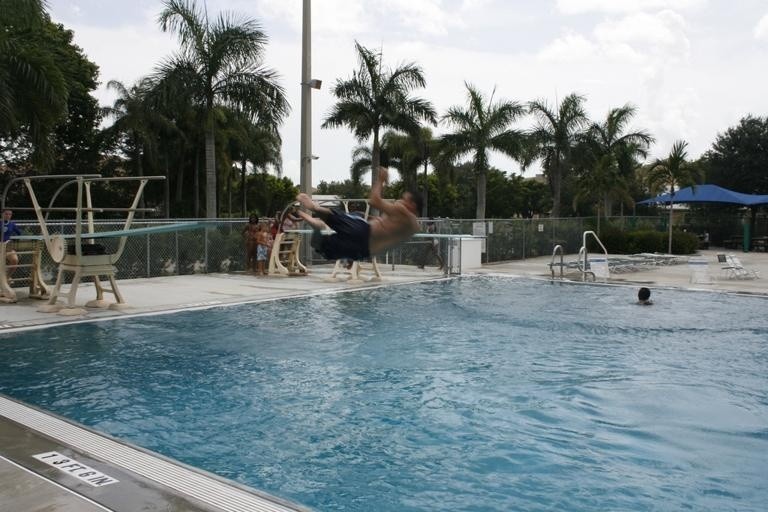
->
[661, 211, 710, 251]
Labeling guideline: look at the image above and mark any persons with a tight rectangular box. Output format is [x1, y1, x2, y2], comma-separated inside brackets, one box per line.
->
[417, 217, 445, 270]
[297, 166, 425, 262]
[240, 202, 304, 277]
[0, 205, 21, 285]
[633, 286, 652, 305]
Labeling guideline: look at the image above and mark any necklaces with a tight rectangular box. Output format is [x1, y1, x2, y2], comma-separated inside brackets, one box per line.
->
[3, 221, 10, 226]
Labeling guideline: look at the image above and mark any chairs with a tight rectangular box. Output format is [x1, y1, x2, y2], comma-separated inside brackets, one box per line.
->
[549, 251, 678, 274]
[716, 253, 760, 281]
